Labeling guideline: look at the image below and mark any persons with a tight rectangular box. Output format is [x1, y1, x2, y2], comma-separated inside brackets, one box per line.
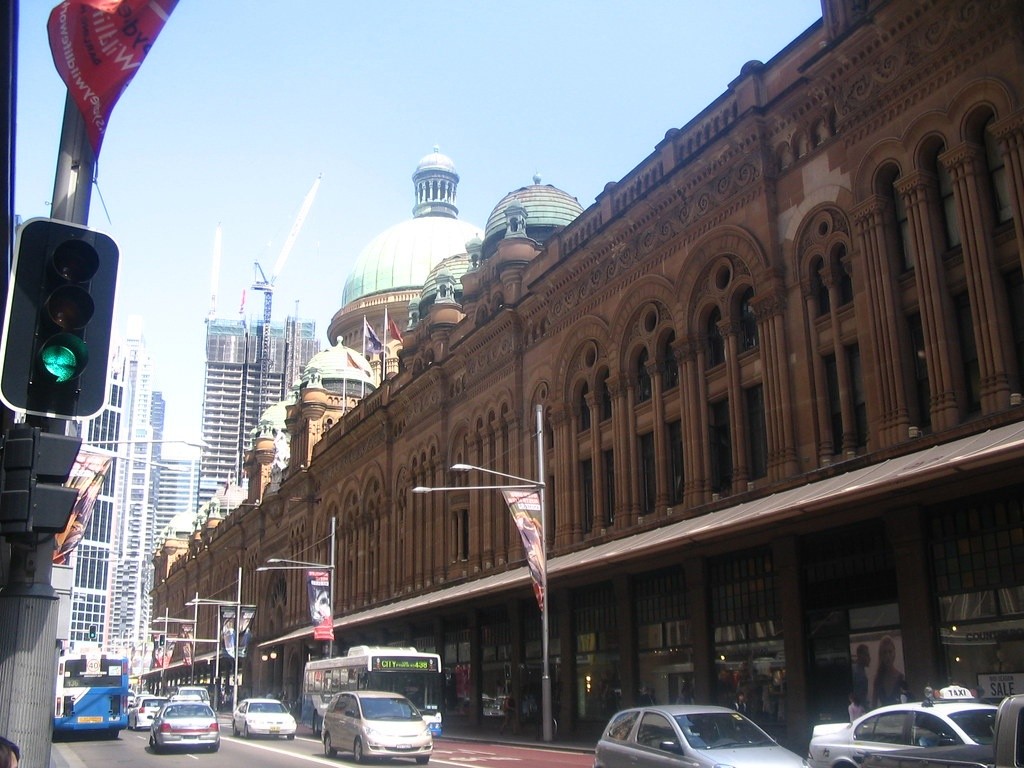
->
[851, 635, 905, 712]
[257, 688, 303, 724]
[898, 681, 915, 704]
[498, 689, 519, 736]
[732, 691, 752, 743]
[847, 691, 867, 736]
[990, 649, 1015, 672]
[0, 735, 20, 768]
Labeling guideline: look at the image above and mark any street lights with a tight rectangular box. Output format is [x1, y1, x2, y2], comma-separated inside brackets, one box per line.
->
[152, 617, 196, 683]
[255, 558, 335, 655]
[185, 598, 242, 710]
[409, 463, 556, 751]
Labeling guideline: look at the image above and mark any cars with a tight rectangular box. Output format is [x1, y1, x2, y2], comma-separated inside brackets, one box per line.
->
[593, 705, 807, 768]
[130, 686, 212, 730]
[232, 698, 297, 741]
[147, 703, 221, 752]
[808, 684, 1001, 768]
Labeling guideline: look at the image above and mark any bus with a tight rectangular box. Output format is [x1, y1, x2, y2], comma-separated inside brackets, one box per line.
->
[53, 652, 129, 739]
[300, 647, 451, 741]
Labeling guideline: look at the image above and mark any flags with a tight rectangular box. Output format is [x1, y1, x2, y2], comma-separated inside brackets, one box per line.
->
[47, 0, 180, 161]
[152, 623, 194, 669]
[500, 488, 546, 614]
[307, 571, 334, 640]
[52, 451, 112, 564]
[347, 311, 403, 377]
[219, 606, 255, 660]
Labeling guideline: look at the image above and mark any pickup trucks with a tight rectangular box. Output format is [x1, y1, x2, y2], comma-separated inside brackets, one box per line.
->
[863, 697, 1024, 768]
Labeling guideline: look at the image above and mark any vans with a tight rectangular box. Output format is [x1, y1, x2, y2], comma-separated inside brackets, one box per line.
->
[320, 689, 432, 765]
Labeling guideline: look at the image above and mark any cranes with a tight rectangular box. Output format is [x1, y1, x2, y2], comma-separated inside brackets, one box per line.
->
[249, 172, 325, 424]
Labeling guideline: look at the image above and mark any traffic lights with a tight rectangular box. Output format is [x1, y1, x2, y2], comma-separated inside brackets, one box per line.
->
[1, 429, 80, 542]
[0, 220, 122, 423]
[160, 635, 165, 646]
[89, 625, 97, 639]
